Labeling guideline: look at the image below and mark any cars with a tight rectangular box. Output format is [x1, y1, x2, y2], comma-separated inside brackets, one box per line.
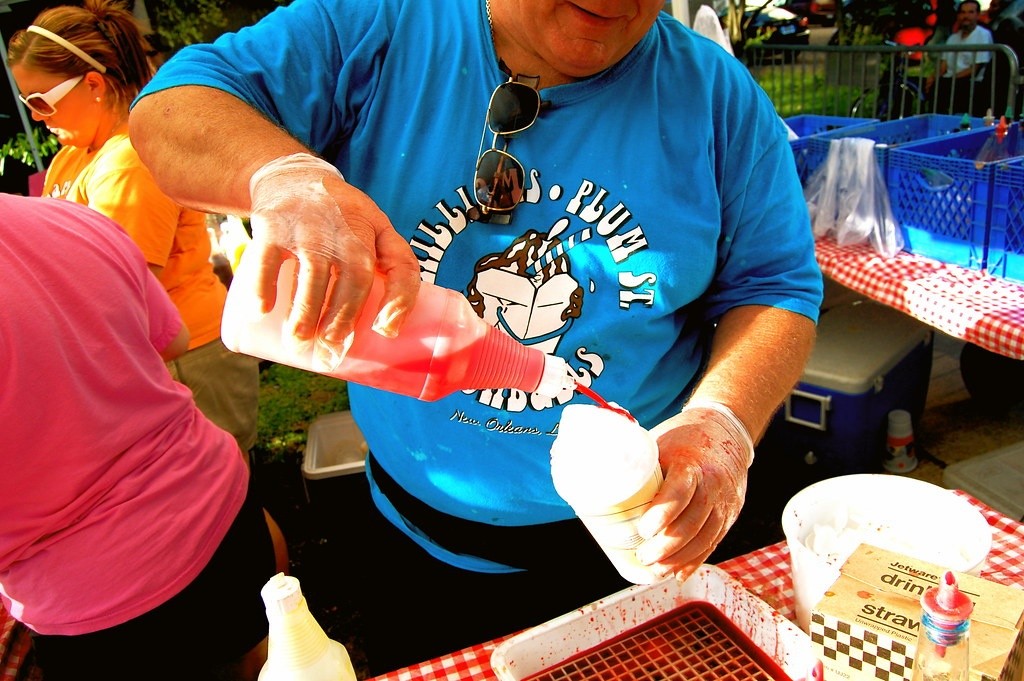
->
[717, 5, 810, 57]
[823, 0, 960, 84]
[986, 0, 1024, 52]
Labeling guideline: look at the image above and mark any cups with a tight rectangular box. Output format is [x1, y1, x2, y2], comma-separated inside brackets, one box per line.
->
[882, 408, 917, 473]
[552, 426, 672, 585]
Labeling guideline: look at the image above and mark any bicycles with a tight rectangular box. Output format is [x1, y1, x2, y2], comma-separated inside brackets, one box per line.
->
[850, 40, 926, 122]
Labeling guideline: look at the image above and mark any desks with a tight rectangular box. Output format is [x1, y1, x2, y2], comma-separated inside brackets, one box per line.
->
[812, 231, 1024, 361]
[364, 487, 1024, 680]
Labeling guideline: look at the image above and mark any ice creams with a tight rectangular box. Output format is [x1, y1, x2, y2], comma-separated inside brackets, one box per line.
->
[550, 400, 657, 512]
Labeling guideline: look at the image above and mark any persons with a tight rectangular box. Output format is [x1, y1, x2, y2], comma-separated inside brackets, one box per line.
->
[926, 0, 1024, 120]
[131, 1, 823, 676]
[1, 0, 289, 681]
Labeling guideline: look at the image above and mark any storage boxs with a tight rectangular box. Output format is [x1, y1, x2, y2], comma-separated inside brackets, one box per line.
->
[782, 112, 1024, 276]
[781, 308, 936, 456]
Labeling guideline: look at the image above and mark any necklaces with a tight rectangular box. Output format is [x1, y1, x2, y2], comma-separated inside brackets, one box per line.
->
[486, 0, 494, 46]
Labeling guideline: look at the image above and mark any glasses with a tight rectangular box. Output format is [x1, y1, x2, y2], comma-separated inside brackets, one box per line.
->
[474, 77, 540, 214]
[19, 75, 85, 117]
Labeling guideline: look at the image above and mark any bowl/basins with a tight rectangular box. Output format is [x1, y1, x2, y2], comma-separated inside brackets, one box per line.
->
[781, 473, 992, 637]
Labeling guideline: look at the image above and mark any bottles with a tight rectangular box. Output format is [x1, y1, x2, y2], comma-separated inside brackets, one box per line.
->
[985, 116, 1010, 162]
[207, 213, 252, 289]
[959, 105, 1013, 130]
[911, 570, 974, 681]
[256, 572, 357, 681]
[221, 238, 574, 402]
